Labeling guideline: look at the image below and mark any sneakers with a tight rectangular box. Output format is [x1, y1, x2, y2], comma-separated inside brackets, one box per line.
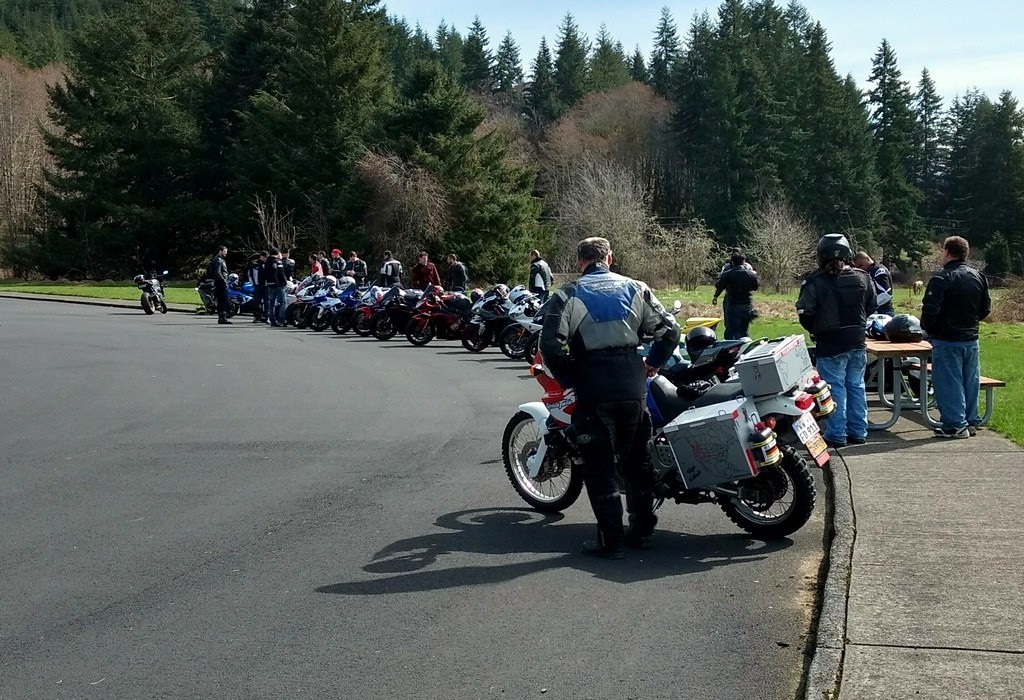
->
[933, 426, 977, 439]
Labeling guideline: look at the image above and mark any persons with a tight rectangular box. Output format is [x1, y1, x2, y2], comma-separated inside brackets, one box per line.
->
[247, 247, 295, 327]
[528, 250, 554, 303]
[212, 246, 233, 324]
[344, 251, 367, 286]
[446, 253, 468, 294]
[538, 237, 681, 560]
[794, 232, 876, 448]
[308, 253, 324, 276]
[382, 250, 403, 287]
[920, 236, 991, 439]
[411, 251, 441, 290]
[328, 249, 346, 279]
[713, 248, 759, 341]
[854, 251, 895, 319]
[317, 251, 331, 276]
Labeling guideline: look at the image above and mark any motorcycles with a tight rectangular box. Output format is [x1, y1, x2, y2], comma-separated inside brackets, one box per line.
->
[133, 271, 169, 315]
[195, 274, 243, 318]
[501, 298, 836, 539]
[286, 264, 550, 366]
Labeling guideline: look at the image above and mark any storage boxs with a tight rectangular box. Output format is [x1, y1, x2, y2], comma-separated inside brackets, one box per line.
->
[663, 396, 769, 492]
[735, 335, 811, 396]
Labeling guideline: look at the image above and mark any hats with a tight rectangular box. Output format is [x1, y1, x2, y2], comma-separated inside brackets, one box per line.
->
[331, 249, 342, 254]
[260, 251, 270, 258]
[270, 248, 280, 256]
[280, 247, 288, 253]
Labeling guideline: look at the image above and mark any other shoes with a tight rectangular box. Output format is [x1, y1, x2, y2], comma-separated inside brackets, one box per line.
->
[218, 319, 233, 324]
[252, 316, 288, 328]
[847, 435, 865, 444]
[821, 437, 847, 447]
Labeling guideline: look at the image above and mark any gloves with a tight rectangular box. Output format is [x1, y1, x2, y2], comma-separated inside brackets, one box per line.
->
[677, 376, 713, 397]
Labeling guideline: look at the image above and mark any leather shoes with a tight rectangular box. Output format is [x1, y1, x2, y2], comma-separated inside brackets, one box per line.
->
[629, 525, 652, 549]
[581, 539, 626, 558]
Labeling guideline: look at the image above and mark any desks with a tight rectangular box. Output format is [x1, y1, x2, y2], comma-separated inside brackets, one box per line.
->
[860, 338, 943, 430]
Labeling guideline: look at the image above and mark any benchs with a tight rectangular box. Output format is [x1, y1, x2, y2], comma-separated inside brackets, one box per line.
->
[909, 364, 1006, 387]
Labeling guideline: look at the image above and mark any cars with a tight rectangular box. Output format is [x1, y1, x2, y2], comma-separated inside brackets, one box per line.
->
[198, 251, 264, 314]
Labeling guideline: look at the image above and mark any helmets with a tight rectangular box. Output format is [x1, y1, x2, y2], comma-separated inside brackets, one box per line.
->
[686, 326, 718, 348]
[338, 276, 356, 290]
[471, 288, 484, 304]
[326, 275, 337, 286]
[495, 284, 510, 300]
[228, 273, 240, 285]
[816, 234, 852, 267]
[433, 285, 444, 296]
[509, 284, 532, 303]
